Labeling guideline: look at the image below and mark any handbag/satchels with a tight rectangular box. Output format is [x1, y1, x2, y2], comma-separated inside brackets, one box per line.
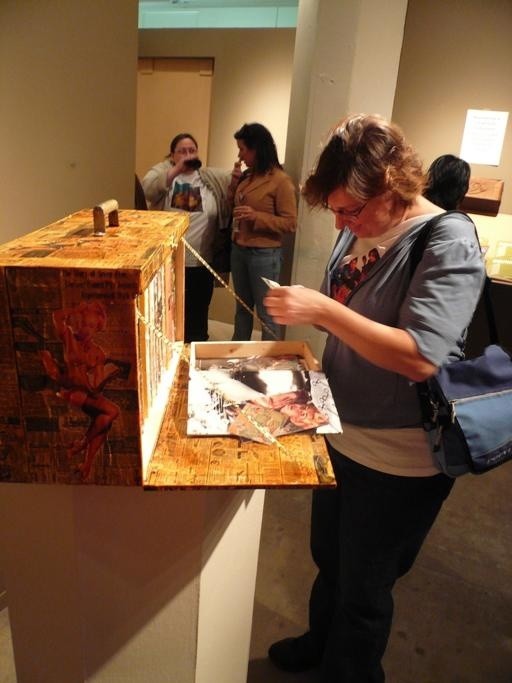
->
[417, 343, 512, 477]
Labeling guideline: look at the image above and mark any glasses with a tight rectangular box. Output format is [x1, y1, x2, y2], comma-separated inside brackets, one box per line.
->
[322, 201, 369, 221]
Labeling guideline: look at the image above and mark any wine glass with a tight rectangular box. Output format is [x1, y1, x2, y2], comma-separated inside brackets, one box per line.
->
[231, 193, 247, 232]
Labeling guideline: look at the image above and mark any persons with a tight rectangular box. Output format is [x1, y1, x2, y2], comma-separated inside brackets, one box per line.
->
[260, 110, 488, 682]
[139, 132, 248, 339]
[419, 151, 472, 210]
[220, 120, 300, 341]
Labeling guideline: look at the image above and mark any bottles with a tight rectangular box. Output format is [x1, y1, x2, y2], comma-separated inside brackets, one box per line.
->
[182, 156, 202, 168]
